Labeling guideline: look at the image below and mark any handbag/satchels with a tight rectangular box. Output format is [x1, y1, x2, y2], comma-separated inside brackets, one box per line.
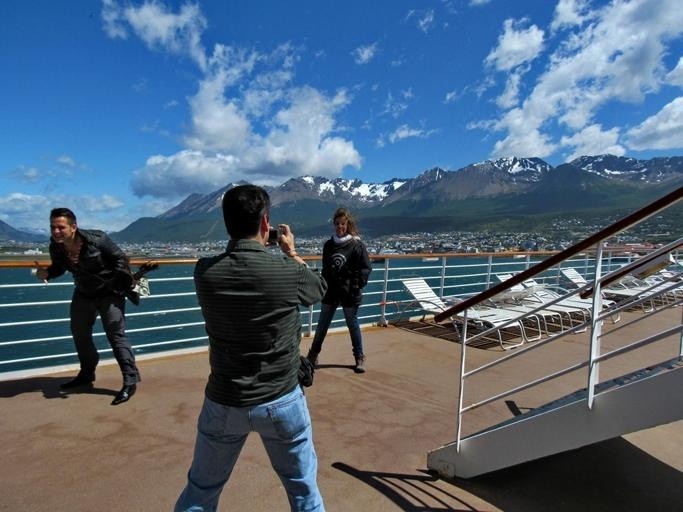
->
[129, 260, 160, 306]
[297, 356, 315, 388]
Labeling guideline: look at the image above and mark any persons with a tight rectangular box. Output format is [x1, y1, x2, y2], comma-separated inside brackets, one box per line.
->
[307, 208, 372, 373]
[36, 207, 141, 405]
[173, 185, 328, 512]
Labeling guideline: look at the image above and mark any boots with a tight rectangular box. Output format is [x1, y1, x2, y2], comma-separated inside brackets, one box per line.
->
[306, 349, 319, 367]
[353, 352, 367, 372]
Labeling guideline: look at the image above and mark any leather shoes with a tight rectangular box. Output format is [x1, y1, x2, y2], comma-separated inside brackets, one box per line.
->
[75, 352, 100, 384]
[111, 383, 137, 404]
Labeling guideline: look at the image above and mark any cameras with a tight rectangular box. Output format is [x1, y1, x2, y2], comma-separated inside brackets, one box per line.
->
[267, 227, 285, 243]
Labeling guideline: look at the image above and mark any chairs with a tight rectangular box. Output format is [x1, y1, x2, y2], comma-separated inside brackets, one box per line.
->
[561, 267, 683, 313]
[388, 273, 621, 351]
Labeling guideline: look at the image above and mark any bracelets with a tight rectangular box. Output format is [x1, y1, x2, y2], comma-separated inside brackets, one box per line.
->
[286, 249, 296, 254]
[288, 253, 299, 258]
